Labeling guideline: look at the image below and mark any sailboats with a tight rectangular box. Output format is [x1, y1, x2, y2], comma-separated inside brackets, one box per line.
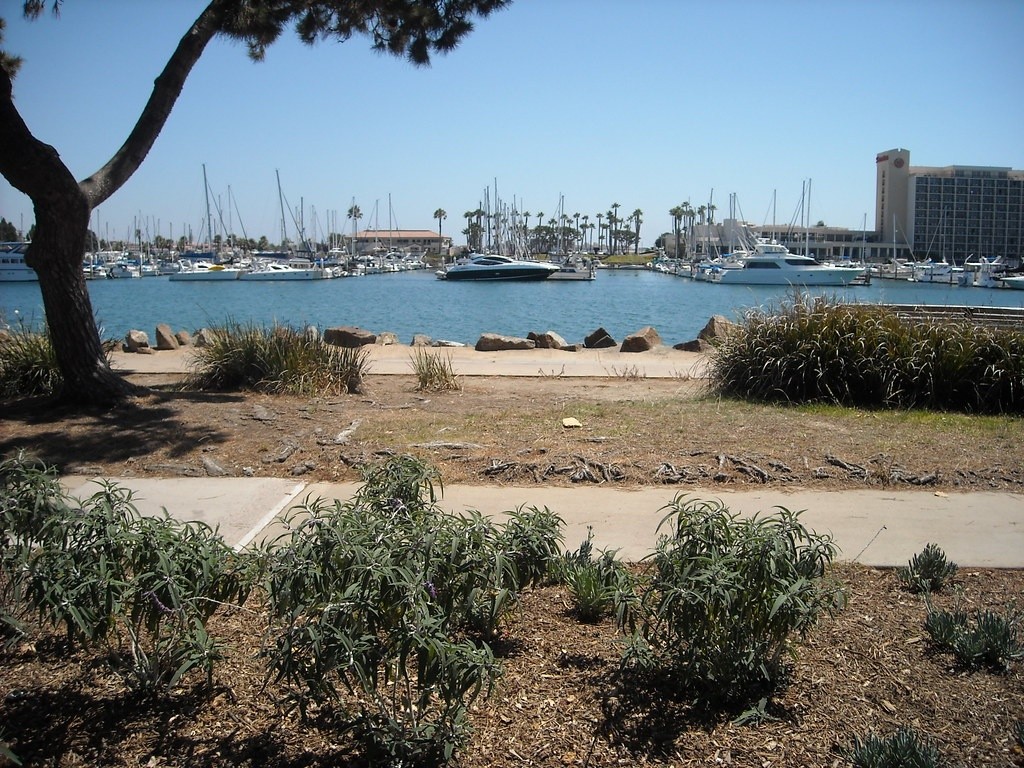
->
[0, 162, 433, 282]
[472, 178, 597, 281]
[646, 178, 1024, 289]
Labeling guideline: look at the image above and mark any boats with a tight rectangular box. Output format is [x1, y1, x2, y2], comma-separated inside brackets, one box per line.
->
[443, 251, 561, 281]
